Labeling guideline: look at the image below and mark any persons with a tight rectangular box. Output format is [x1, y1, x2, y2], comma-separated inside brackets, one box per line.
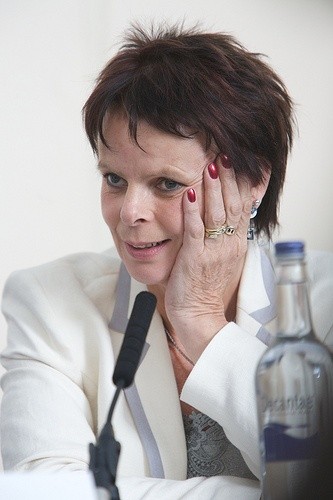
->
[2, 19, 333, 500]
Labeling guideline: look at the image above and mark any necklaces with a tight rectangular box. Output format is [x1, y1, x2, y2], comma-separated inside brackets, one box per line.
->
[165, 328, 196, 368]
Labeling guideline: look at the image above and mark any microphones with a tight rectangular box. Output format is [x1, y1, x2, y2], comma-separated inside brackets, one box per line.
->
[107, 291, 158, 425]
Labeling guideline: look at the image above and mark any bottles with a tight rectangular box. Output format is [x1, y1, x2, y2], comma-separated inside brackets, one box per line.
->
[254, 242, 333, 500]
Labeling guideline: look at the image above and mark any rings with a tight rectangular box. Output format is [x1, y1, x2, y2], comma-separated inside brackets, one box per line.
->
[227, 226, 239, 235]
[206, 224, 226, 239]
